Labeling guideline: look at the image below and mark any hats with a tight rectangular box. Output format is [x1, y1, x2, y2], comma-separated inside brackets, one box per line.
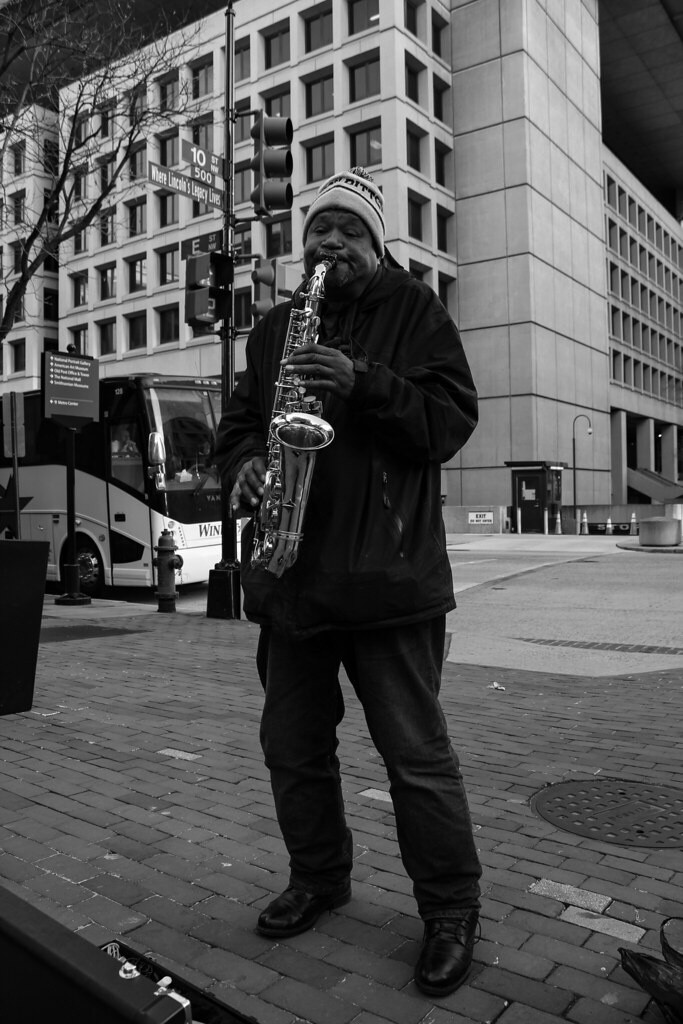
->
[303, 165, 385, 259]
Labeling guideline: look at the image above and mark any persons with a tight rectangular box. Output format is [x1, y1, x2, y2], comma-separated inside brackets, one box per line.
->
[111, 430, 139, 458]
[207, 165, 484, 1000]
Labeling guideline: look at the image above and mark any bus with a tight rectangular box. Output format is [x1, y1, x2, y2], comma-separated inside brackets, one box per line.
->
[0, 372, 252, 599]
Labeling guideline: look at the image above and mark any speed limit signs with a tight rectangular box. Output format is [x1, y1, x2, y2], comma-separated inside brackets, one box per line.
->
[182, 139, 223, 178]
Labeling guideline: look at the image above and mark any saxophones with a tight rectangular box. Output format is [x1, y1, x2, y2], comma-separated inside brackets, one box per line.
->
[250, 256, 339, 586]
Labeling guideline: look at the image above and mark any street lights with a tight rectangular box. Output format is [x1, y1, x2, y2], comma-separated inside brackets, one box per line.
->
[572, 414, 593, 518]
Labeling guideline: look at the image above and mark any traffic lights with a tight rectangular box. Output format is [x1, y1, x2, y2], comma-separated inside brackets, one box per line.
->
[187, 251, 233, 327]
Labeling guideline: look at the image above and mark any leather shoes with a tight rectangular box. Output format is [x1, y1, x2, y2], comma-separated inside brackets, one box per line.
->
[257, 877, 351, 937]
[417, 910, 481, 995]
